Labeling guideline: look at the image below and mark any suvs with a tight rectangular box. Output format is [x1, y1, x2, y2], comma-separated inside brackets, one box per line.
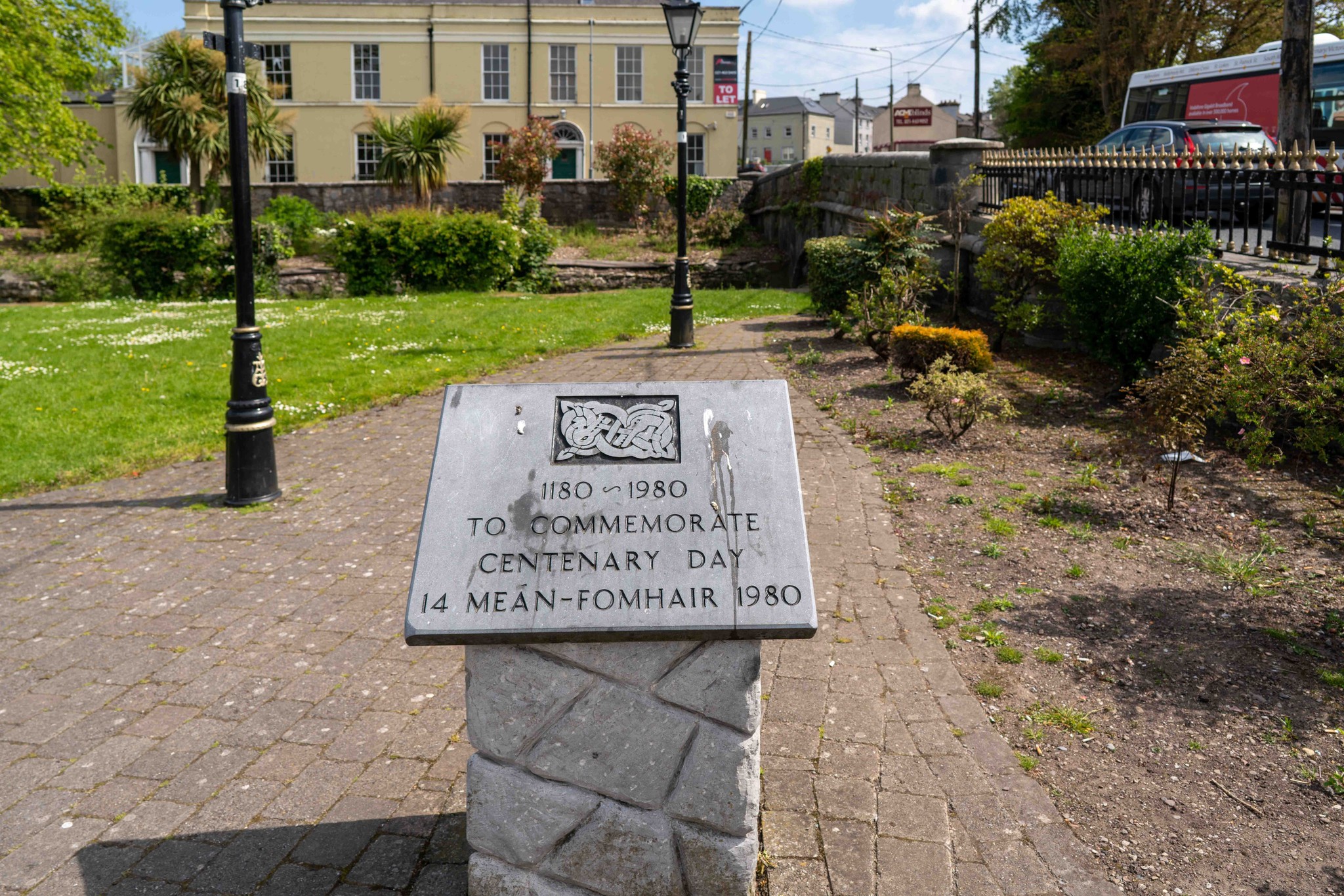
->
[1055, 117, 1278, 229]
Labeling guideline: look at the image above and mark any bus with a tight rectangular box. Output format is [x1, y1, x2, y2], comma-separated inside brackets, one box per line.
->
[1119, 32, 1344, 216]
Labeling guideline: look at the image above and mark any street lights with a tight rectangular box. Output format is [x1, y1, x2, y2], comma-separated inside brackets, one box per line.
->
[661, 1, 705, 346]
[869, 47, 895, 151]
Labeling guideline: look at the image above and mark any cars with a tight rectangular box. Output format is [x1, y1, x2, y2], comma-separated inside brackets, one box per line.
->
[996, 149, 1062, 201]
